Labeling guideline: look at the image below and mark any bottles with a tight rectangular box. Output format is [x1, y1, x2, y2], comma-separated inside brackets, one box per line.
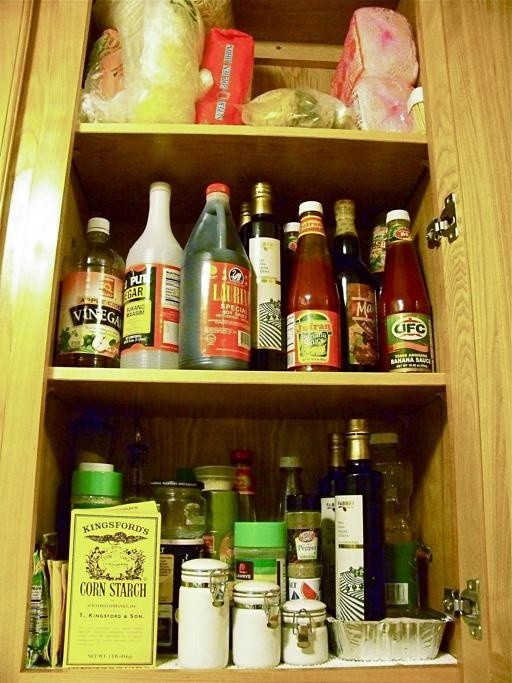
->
[238, 182, 435, 373]
[54, 182, 183, 369]
[67, 443, 153, 508]
[196, 418, 419, 620]
[178, 557, 329, 668]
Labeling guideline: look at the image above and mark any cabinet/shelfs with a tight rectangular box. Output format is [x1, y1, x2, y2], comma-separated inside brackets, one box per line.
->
[18, 0, 462, 673]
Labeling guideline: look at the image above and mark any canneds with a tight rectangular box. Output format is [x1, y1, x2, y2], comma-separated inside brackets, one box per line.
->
[145, 477, 208, 539]
